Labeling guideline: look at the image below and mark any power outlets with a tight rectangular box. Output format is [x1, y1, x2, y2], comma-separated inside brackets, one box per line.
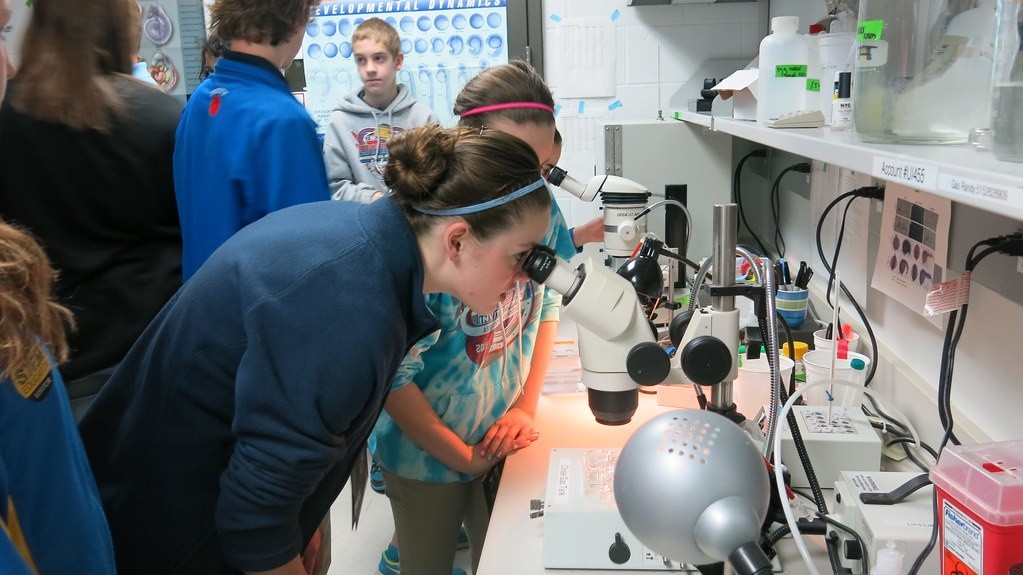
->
[874, 181, 886, 212]
[762, 144, 771, 167]
[1014, 224, 1023, 272]
[803, 158, 812, 184]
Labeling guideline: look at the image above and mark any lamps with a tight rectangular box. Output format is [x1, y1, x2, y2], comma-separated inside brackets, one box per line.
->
[616, 233, 806, 407]
[613, 407, 783, 575]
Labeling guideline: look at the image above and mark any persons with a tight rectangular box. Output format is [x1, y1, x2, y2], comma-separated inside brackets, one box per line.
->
[324, 17, 438, 200]
[199, 35, 231, 83]
[368, 62, 575, 575]
[174, 0, 331, 284]
[0, 0, 117, 575]
[78, 126, 550, 575]
[381, 128, 603, 575]
[0, 0, 180, 379]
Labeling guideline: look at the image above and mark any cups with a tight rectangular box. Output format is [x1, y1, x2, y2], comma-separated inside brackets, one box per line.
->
[732, 327, 871, 434]
[850, 1, 999, 144]
[990, 0, 1023, 162]
[775, 284, 809, 328]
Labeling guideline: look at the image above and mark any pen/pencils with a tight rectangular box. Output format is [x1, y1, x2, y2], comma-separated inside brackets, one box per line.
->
[784, 262, 792, 291]
[794, 261, 814, 291]
[776, 264, 785, 290]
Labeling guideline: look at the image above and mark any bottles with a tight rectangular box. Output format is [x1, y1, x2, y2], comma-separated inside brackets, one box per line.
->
[757, 17, 854, 132]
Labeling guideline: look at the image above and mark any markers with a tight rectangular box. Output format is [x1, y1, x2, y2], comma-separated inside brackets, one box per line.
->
[825, 323, 833, 339]
[838, 318, 845, 339]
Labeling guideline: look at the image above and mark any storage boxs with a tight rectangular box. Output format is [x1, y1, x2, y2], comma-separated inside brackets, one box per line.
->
[927, 435, 1023, 575]
[711, 63, 760, 120]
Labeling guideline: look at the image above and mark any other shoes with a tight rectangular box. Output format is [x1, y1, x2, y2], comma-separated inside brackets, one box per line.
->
[369, 458, 384, 491]
[453, 525, 470, 549]
[378, 547, 467, 575]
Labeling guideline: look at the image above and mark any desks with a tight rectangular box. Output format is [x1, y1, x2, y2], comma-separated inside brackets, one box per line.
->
[476, 261, 924, 575]
